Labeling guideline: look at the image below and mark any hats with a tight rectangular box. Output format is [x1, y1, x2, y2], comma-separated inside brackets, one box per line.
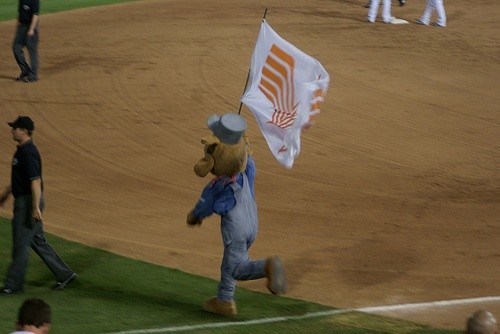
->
[8, 116, 34, 130]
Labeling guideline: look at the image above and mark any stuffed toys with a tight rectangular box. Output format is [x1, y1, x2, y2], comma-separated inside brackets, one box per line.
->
[185, 109, 290, 319]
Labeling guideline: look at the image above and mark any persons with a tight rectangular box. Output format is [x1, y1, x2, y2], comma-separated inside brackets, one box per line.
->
[363, 0, 407, 25]
[414, 0, 448, 27]
[463, 309, 498, 334]
[11, 0, 43, 83]
[0, 114, 79, 296]
[9, 297, 52, 334]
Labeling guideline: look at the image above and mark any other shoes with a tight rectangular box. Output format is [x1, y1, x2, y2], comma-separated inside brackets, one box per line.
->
[51, 270, 80, 290]
[0, 286, 24, 295]
[16, 72, 37, 82]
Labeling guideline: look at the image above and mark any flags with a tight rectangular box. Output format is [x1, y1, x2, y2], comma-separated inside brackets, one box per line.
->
[242, 19, 333, 170]
